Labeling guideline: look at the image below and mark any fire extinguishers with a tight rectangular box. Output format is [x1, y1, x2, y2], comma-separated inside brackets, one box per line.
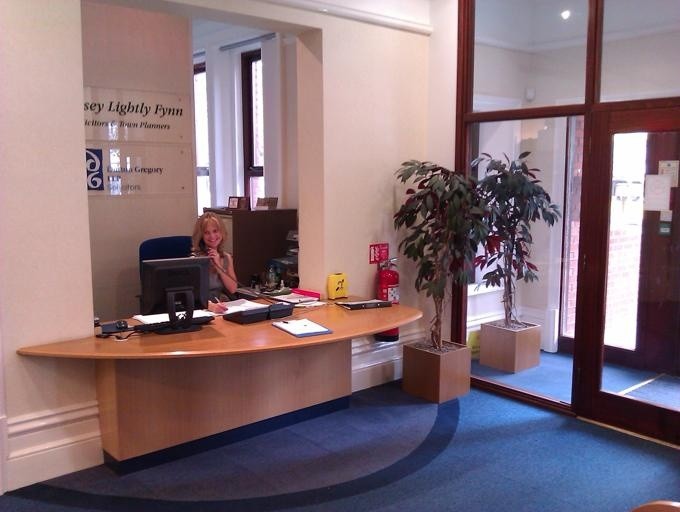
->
[373, 257, 400, 342]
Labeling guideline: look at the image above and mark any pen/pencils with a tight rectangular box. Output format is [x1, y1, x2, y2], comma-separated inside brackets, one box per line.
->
[215, 296, 220, 303]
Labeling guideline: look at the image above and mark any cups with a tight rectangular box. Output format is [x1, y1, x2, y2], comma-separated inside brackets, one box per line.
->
[108, 174, 122, 196]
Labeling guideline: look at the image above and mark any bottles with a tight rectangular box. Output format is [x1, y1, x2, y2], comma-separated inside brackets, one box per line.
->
[264, 261, 281, 285]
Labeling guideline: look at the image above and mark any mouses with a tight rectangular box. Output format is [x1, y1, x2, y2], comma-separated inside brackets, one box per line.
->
[116, 321, 127, 329]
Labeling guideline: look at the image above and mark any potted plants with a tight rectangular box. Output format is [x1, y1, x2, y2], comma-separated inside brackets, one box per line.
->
[393, 158, 501, 405]
[470, 151, 564, 375]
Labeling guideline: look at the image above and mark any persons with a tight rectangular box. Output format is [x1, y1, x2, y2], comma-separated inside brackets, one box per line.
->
[189, 212, 238, 314]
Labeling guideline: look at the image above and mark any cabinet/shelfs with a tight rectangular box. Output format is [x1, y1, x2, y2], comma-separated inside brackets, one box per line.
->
[204, 206, 298, 288]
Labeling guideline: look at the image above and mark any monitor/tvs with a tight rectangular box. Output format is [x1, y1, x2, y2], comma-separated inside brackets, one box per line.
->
[141, 256, 209, 335]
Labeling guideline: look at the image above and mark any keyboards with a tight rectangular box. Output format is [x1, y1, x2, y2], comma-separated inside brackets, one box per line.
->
[134, 316, 214, 331]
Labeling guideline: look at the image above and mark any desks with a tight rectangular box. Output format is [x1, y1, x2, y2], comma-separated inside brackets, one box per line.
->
[18, 297, 423, 462]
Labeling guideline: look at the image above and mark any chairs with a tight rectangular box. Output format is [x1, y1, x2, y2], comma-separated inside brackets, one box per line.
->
[138, 235, 194, 261]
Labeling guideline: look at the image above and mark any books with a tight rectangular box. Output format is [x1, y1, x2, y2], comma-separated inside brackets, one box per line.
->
[269, 293, 327, 308]
[272, 318, 332, 338]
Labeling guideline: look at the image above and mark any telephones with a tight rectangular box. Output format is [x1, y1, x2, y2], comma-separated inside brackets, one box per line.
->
[199, 238, 211, 255]
